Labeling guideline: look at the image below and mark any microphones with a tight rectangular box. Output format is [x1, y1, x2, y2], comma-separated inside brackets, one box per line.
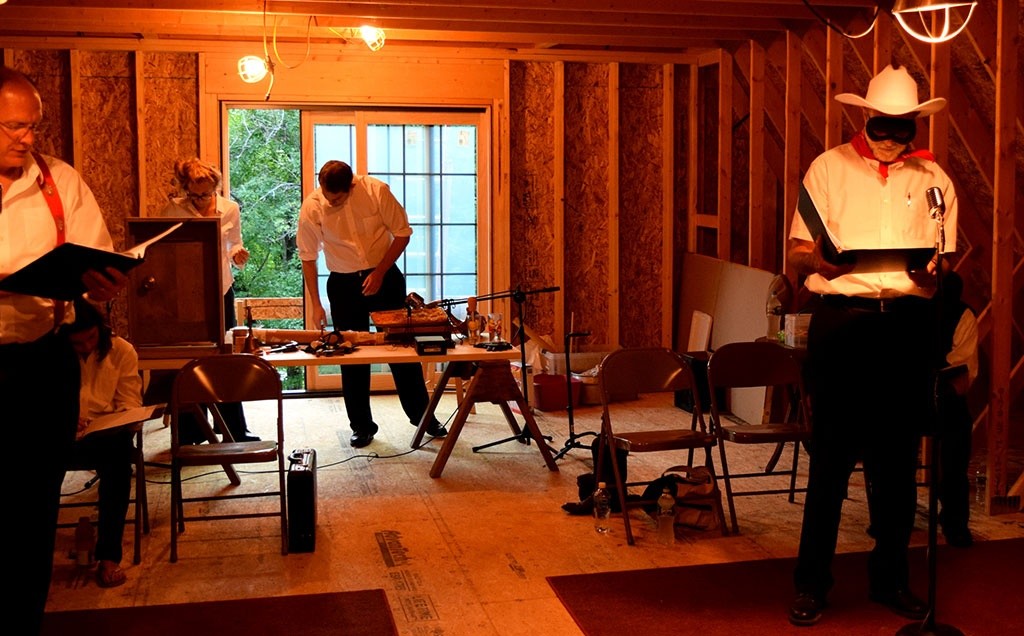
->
[926, 186, 946, 219]
[405, 292, 424, 308]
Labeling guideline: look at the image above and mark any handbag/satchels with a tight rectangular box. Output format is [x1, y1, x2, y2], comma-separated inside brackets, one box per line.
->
[641, 465, 720, 531]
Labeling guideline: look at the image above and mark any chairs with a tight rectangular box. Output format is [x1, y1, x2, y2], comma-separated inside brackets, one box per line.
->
[594, 342, 810, 546]
[169, 353, 287, 563]
[68, 367, 150, 566]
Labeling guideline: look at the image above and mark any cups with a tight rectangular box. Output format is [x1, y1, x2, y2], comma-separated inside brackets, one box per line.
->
[488, 313, 502, 342]
[466, 315, 481, 344]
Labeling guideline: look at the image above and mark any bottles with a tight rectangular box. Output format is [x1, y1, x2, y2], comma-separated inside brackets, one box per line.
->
[592, 482, 610, 533]
[657, 485, 674, 546]
[74, 516, 96, 568]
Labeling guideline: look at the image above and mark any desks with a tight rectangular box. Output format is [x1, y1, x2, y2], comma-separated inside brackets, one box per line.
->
[136, 332, 559, 479]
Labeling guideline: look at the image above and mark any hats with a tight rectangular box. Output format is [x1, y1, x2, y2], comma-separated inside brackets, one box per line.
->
[834, 62, 946, 118]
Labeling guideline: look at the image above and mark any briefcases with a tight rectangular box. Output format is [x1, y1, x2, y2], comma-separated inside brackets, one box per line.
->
[287, 448, 318, 552]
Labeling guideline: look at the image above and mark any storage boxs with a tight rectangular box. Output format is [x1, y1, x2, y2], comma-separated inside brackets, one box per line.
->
[533, 348, 608, 412]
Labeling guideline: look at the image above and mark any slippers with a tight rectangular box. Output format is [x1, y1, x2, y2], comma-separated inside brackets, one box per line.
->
[98, 561, 126, 587]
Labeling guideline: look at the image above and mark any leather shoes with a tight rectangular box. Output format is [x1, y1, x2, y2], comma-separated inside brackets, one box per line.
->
[873, 583, 926, 619]
[789, 589, 823, 623]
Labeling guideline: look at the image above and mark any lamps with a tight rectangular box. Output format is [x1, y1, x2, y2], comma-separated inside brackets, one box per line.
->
[891, 0, 979, 42]
[238, 0, 387, 102]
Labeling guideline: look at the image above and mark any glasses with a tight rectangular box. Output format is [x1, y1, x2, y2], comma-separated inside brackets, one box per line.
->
[187, 191, 212, 201]
[0, 122, 48, 137]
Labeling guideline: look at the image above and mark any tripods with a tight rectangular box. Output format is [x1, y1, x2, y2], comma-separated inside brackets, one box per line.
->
[426, 286, 564, 458]
[543, 331, 598, 466]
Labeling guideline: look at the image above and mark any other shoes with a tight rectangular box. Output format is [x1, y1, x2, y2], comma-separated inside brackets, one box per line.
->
[943, 523, 972, 548]
[411, 415, 447, 436]
[237, 433, 261, 440]
[351, 427, 371, 446]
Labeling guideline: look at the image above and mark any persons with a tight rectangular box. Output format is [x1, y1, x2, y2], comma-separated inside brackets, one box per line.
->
[789, 65, 960, 625]
[295, 161, 447, 446]
[68, 292, 143, 587]
[157, 158, 260, 443]
[0, 65, 128, 615]
[921, 270, 979, 548]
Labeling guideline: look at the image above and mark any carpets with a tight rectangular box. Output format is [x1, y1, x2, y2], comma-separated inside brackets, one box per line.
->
[44, 589, 398, 636]
[546, 538, 1024, 636]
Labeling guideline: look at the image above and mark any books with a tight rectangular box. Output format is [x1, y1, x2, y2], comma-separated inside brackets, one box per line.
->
[0, 222, 182, 303]
